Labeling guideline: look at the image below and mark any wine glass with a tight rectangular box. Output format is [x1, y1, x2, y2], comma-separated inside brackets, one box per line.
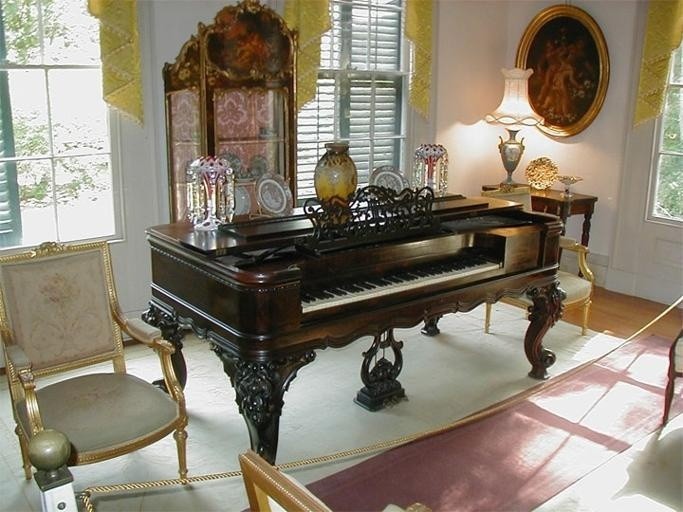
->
[556, 176, 583, 198]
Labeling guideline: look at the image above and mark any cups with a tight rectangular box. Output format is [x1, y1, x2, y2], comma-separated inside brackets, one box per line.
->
[260, 127, 277, 139]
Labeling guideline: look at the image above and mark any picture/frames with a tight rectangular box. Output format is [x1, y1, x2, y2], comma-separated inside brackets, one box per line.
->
[515, 3, 613, 139]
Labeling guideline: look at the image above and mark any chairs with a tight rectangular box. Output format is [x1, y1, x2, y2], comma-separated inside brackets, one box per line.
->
[0, 241, 190, 481]
[660, 332, 683, 427]
[238, 451, 438, 512]
[482, 183, 595, 336]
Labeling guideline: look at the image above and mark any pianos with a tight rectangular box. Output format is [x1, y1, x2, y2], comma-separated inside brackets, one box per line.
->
[141, 186, 567, 466]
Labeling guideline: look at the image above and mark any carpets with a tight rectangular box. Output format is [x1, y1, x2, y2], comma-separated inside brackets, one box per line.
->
[0, 297, 681, 512]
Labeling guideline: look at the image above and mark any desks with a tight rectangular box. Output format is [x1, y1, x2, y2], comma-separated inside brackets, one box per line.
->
[480, 183, 599, 278]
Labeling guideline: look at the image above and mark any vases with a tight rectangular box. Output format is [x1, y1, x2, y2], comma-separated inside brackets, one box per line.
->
[314, 144, 358, 223]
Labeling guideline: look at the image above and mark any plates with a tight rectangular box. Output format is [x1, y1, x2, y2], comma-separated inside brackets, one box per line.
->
[369, 164, 411, 193]
[220, 151, 293, 218]
[526, 156, 558, 191]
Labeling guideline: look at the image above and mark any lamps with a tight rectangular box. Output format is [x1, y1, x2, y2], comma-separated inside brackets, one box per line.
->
[486, 67, 545, 189]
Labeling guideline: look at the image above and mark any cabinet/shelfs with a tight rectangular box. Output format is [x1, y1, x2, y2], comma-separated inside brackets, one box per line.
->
[161, 0, 299, 223]
